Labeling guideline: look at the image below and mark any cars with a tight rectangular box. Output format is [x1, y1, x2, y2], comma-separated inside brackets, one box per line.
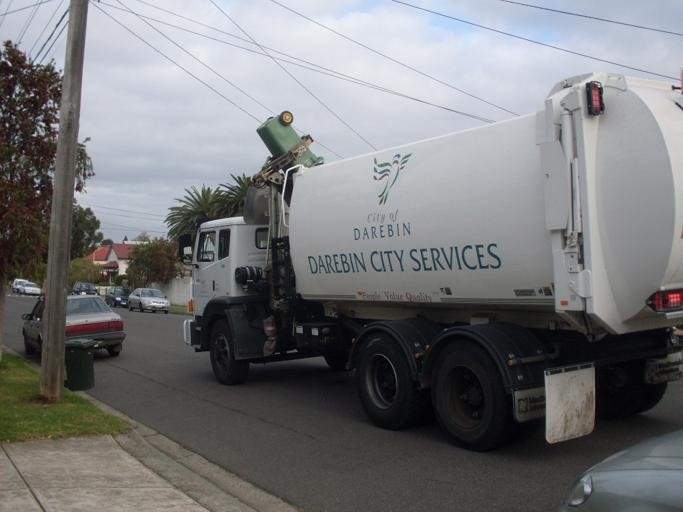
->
[559, 429, 683, 512]
[72, 281, 170, 314]
[12, 279, 42, 295]
[22, 294, 126, 356]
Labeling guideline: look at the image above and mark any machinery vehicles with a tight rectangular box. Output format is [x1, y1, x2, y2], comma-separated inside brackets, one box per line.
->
[179, 71, 683, 452]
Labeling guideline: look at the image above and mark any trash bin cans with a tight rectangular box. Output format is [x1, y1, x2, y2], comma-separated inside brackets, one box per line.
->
[256, 111, 323, 177]
[64, 339, 96, 391]
